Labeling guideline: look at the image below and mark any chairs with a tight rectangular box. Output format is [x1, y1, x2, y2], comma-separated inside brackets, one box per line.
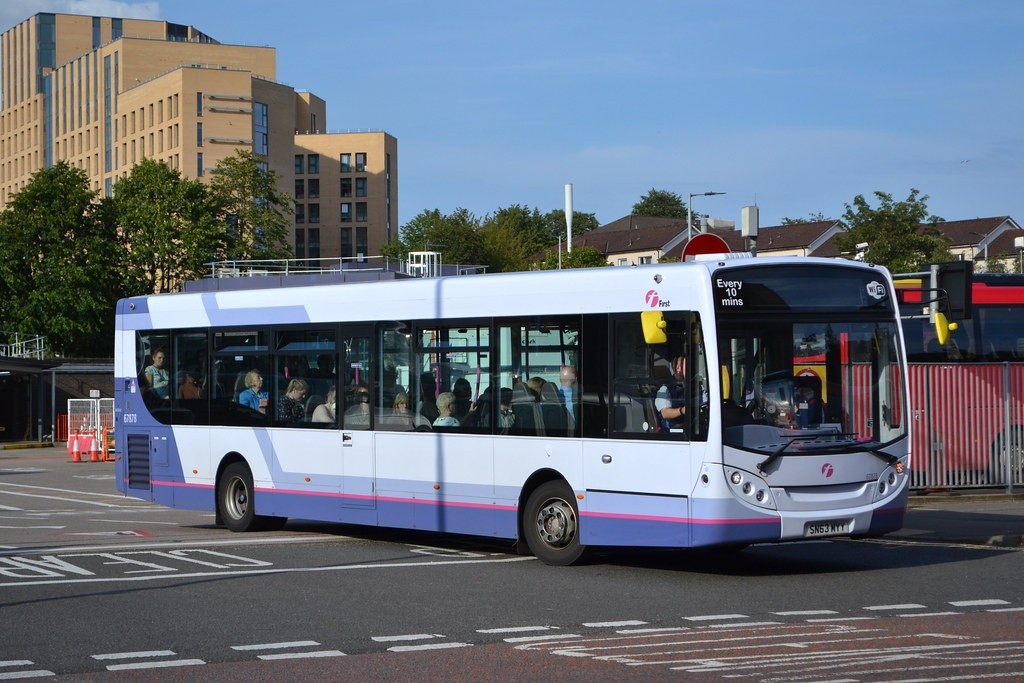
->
[145, 351, 707, 437]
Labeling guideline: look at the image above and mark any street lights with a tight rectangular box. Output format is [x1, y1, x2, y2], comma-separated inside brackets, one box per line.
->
[968, 231, 988, 270]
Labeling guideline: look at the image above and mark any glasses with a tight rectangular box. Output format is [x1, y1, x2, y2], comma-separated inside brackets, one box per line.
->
[390, 375, 398, 380]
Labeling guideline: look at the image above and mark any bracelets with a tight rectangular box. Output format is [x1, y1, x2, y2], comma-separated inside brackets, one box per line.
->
[679, 407, 684, 416]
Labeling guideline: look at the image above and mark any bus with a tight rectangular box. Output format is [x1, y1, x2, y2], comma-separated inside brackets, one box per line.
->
[722, 270, 1024, 489]
[111, 251, 961, 568]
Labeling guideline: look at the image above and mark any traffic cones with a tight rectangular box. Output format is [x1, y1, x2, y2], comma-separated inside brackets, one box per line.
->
[89, 434, 99, 461]
[72, 432, 81, 461]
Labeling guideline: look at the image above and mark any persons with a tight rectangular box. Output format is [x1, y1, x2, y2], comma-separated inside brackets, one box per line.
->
[654, 355, 708, 433]
[144, 345, 577, 429]
[746, 377, 779, 420]
